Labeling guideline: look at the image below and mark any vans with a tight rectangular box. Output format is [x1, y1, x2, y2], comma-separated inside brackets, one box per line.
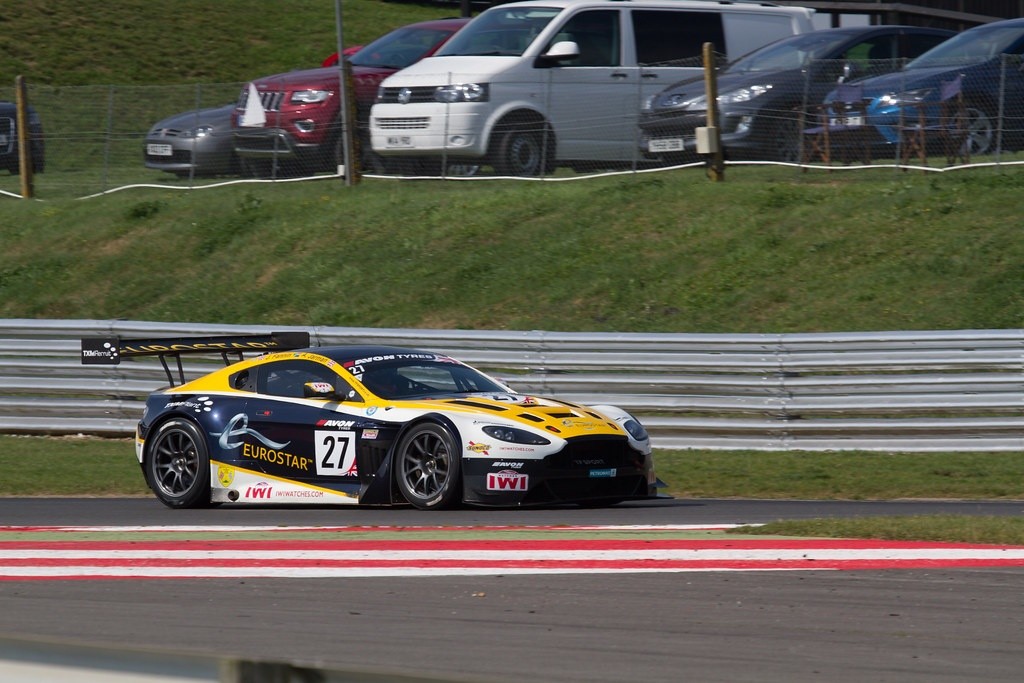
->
[371, 1, 817, 178]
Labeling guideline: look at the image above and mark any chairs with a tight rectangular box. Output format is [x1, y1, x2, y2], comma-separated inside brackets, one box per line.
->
[550, 33, 586, 66]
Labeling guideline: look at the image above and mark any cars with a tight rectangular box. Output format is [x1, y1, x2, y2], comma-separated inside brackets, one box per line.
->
[80, 331, 675, 510]
[230, 16, 547, 178]
[141, 103, 243, 179]
[0, 101, 45, 174]
[635, 25, 961, 162]
[805, 19, 1024, 162]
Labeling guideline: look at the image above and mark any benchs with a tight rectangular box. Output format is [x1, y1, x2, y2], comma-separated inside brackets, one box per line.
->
[891, 101, 975, 167]
[789, 100, 876, 166]
